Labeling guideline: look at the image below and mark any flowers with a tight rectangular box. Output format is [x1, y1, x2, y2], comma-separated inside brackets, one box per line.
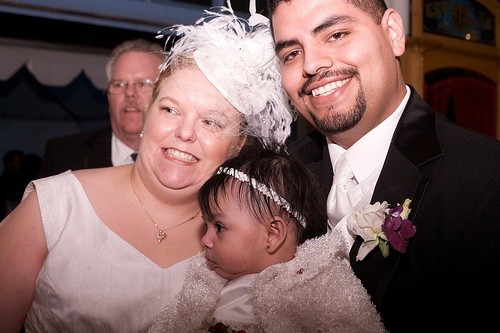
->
[347, 198, 417, 260]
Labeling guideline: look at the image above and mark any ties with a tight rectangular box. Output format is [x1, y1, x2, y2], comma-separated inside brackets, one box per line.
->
[327, 153, 356, 225]
[131, 154, 138, 162]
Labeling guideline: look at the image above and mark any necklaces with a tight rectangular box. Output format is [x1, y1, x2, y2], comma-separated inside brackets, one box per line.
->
[128, 169, 203, 243]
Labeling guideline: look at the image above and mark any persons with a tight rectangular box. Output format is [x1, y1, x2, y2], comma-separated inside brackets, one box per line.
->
[267, 0, 500, 333]
[198, 151, 389, 333]
[0, 0, 300, 333]
[0, 38, 169, 221]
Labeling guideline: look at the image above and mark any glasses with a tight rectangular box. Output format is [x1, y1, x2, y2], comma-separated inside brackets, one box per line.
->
[105, 79, 154, 94]
